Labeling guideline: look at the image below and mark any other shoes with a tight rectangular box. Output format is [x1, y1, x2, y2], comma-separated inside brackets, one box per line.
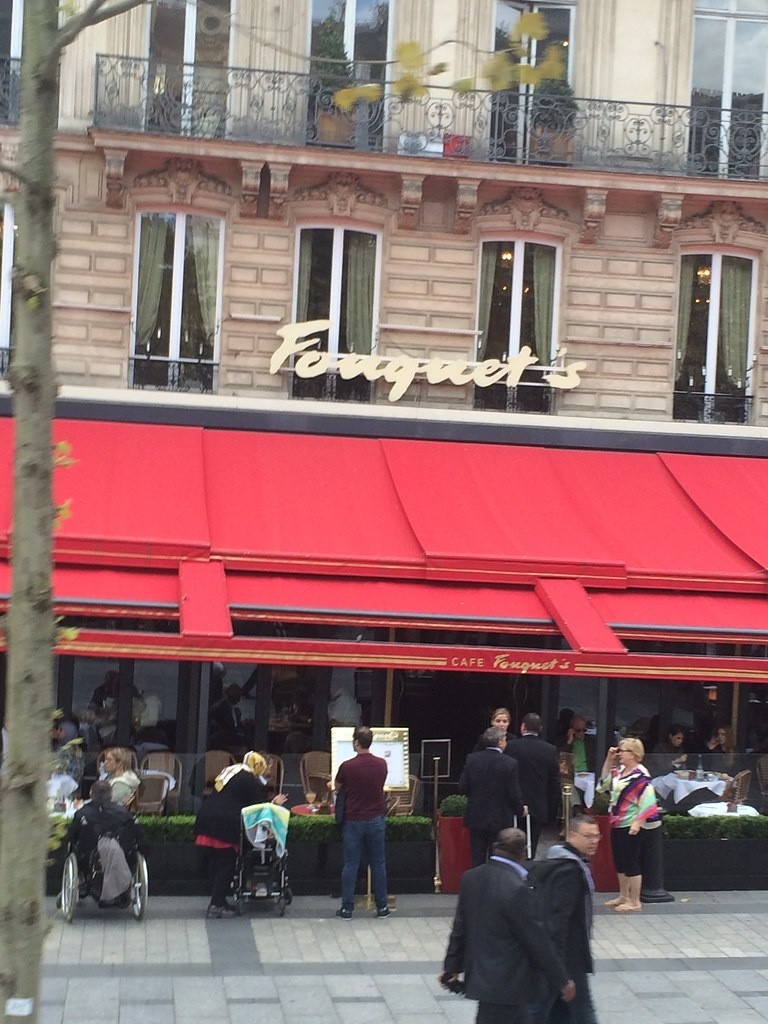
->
[208, 904, 234, 918]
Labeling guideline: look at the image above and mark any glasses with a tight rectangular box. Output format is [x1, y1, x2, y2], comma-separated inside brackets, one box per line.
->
[618, 748, 632, 752]
[574, 728, 587, 733]
[574, 832, 603, 843]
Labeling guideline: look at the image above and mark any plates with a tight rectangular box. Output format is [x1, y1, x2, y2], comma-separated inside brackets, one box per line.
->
[673, 769, 695, 773]
[703, 771, 712, 773]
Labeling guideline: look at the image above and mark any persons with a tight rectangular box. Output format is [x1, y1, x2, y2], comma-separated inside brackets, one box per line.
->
[595, 737, 661, 911]
[503, 713, 561, 860]
[88, 670, 147, 728]
[526, 814, 603, 1024]
[67, 781, 145, 874]
[555, 715, 595, 773]
[335, 725, 392, 921]
[103, 747, 141, 804]
[472, 707, 517, 753]
[215, 683, 243, 753]
[458, 727, 528, 869]
[437, 827, 575, 1024]
[192, 751, 287, 919]
[654, 723, 730, 775]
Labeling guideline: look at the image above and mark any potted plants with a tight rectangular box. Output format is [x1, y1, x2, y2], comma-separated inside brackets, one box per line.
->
[304, 14, 363, 148]
[529, 76, 579, 163]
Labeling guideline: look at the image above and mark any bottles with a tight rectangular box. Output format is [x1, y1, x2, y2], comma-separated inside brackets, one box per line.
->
[696, 754, 704, 781]
[707, 773, 713, 781]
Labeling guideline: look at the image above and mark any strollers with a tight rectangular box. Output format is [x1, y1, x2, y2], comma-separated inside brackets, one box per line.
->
[233, 802, 295, 917]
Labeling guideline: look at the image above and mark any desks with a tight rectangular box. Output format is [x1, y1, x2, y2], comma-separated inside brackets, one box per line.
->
[291, 797, 355, 821]
[688, 802, 759, 823]
[100, 768, 176, 793]
[659, 760, 745, 798]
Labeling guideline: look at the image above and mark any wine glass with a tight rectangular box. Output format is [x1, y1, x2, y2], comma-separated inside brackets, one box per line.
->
[305, 793, 317, 809]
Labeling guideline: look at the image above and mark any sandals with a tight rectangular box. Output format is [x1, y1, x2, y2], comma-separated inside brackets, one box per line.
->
[604, 898, 627, 906]
[615, 902, 642, 912]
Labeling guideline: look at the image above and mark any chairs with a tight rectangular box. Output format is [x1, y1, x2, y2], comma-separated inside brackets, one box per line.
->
[94, 741, 768, 845]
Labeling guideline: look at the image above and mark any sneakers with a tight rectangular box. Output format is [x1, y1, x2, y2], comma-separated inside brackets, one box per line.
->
[377, 906, 392, 918]
[336, 908, 352, 921]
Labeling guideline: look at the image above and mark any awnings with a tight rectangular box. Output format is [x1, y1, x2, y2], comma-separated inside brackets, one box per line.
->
[0, 415, 768, 683]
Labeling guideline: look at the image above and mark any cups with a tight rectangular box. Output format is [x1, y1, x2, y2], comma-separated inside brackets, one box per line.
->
[320, 791, 328, 802]
[55, 804, 66, 812]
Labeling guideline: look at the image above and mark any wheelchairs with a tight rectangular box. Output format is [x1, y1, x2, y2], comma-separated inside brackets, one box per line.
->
[60, 825, 149, 922]
